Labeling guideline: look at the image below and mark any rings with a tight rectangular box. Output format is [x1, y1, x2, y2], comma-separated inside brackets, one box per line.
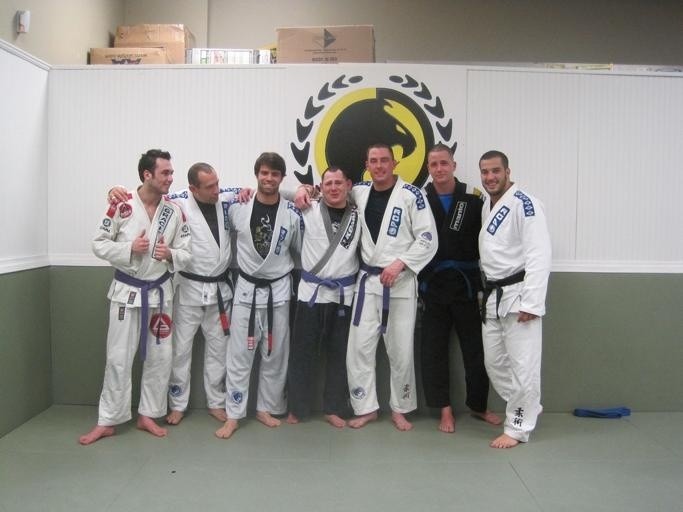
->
[112, 196, 116, 200]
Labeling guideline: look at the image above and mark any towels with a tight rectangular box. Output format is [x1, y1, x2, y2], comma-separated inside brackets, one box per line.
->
[573, 406, 631, 418]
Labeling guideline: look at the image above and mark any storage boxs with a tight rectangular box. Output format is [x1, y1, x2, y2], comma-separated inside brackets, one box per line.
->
[114, 24, 196, 64]
[275, 25, 376, 63]
[90, 47, 172, 65]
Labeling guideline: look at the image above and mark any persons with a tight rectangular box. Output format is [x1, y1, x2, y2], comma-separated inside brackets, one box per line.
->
[107, 162, 245, 425]
[477, 151, 552, 449]
[293, 142, 439, 431]
[215, 152, 304, 439]
[79, 149, 194, 445]
[420, 144, 503, 433]
[237, 166, 361, 428]
